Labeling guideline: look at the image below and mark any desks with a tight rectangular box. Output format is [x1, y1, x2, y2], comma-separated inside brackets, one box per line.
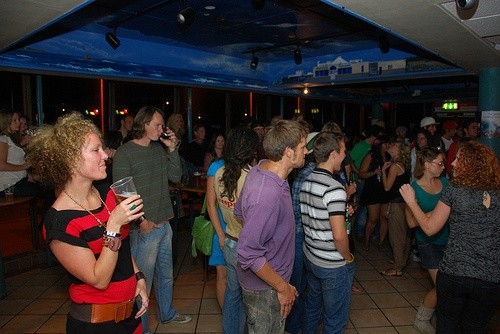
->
[0, 196, 40, 253]
[169, 179, 208, 231]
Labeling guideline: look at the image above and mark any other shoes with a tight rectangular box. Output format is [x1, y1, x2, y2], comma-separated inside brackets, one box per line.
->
[170, 312, 192, 324]
[378, 244, 386, 251]
[363, 244, 369, 251]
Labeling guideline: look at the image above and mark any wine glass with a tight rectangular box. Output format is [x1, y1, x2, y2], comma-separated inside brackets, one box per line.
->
[158, 126, 181, 148]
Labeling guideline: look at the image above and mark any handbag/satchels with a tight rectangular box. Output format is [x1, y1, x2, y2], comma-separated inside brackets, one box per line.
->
[191, 196, 214, 257]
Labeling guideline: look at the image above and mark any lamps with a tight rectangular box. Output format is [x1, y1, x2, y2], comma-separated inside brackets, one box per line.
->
[294, 46, 302, 65]
[250, 51, 258, 69]
[377, 37, 389, 54]
[177, 0, 194, 24]
[105, 24, 120, 48]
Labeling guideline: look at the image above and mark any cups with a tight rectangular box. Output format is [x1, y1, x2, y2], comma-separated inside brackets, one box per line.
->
[193, 167, 201, 176]
[110, 176, 144, 223]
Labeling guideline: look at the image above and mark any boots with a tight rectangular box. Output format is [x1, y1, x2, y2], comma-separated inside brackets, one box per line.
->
[413, 300, 435, 334]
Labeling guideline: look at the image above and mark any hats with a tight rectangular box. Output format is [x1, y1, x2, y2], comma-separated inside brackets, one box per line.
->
[305, 132, 321, 155]
[420, 117, 440, 127]
[396, 126, 406, 131]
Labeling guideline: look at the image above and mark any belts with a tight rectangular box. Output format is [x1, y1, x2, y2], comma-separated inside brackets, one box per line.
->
[70, 299, 141, 323]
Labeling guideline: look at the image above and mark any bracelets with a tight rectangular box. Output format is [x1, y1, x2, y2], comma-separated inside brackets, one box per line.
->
[102, 237, 122, 252]
[136, 215, 146, 224]
[346, 253, 354, 265]
[102, 231, 122, 238]
[135, 272, 147, 283]
[370, 172, 372, 177]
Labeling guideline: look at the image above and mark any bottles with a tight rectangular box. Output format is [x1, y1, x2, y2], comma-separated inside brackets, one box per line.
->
[344, 203, 351, 234]
[349, 171, 354, 186]
[376, 164, 381, 182]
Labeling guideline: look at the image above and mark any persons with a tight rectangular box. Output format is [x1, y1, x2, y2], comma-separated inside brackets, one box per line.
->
[399, 140, 500, 334]
[405, 146, 450, 334]
[0, 110, 500, 276]
[23, 110, 149, 334]
[205, 115, 308, 334]
[286, 132, 356, 334]
[112, 105, 192, 334]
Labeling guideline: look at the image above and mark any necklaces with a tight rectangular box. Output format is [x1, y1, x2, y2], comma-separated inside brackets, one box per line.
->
[62, 188, 111, 227]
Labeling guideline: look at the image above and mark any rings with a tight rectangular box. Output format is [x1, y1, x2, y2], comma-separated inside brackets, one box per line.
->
[129, 204, 137, 210]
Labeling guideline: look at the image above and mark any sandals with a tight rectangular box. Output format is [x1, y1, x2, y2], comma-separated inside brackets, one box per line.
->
[379, 269, 403, 277]
[388, 259, 394, 265]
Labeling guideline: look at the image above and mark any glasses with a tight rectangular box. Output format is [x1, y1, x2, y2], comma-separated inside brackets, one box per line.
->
[428, 161, 445, 167]
